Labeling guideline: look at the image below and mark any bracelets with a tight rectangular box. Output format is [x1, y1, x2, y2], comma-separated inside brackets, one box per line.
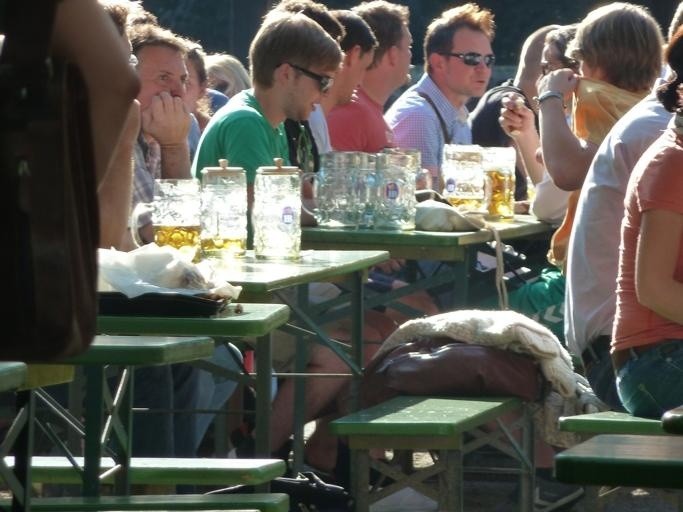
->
[534, 91, 568, 110]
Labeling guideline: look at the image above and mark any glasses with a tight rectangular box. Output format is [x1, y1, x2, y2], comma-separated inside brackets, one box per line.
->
[541, 61, 551, 75]
[433, 49, 496, 68]
[277, 61, 334, 94]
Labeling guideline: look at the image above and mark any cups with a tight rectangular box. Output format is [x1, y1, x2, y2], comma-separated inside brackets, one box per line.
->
[253, 174, 302, 261]
[132, 179, 202, 260]
[200, 174, 248, 256]
[299, 152, 365, 227]
[484, 148, 518, 218]
[440, 145, 483, 191]
[374, 148, 421, 228]
[443, 175, 489, 216]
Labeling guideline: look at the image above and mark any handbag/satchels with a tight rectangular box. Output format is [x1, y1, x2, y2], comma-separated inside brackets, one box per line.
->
[268, 471, 347, 512]
[370, 333, 541, 402]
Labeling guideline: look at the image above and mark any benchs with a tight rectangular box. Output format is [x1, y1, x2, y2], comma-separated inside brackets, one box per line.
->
[1, 492, 291, 512]
[4, 455, 286, 490]
[329, 391, 539, 510]
[556, 409, 667, 434]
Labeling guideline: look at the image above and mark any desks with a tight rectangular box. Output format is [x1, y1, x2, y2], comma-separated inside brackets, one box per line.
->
[300, 201, 554, 416]
[10, 330, 210, 495]
[59, 304, 290, 460]
[92, 249, 390, 467]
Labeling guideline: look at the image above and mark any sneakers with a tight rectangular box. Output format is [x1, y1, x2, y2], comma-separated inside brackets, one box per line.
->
[510, 486, 583, 512]
[228, 435, 291, 476]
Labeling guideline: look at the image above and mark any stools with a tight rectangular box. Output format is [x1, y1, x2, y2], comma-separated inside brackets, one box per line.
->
[551, 434, 683, 511]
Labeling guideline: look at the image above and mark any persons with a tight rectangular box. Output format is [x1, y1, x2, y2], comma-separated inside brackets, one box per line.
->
[127, 24, 244, 457]
[0, 0, 142, 362]
[564, 3, 683, 415]
[499, 22, 581, 224]
[470, 26, 558, 199]
[102, 0, 159, 23]
[477, 4, 662, 511]
[203, 54, 251, 98]
[329, 2, 411, 152]
[275, 0, 399, 339]
[99, 8, 198, 495]
[190, 14, 381, 477]
[610, 28, 683, 419]
[384, 6, 495, 196]
[308, 9, 439, 325]
[176, 36, 212, 156]
